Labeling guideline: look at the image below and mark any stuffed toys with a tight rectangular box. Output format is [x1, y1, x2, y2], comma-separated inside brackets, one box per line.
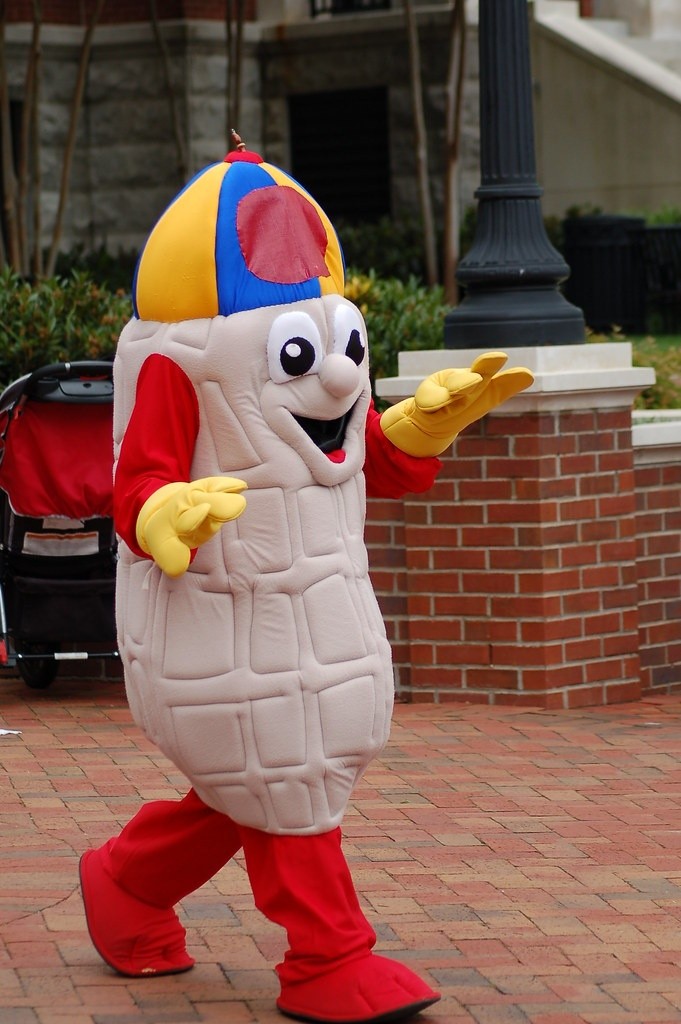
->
[80, 153, 536, 1024]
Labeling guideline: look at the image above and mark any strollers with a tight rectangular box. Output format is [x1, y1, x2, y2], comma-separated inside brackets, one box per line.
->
[3, 357, 123, 688]
